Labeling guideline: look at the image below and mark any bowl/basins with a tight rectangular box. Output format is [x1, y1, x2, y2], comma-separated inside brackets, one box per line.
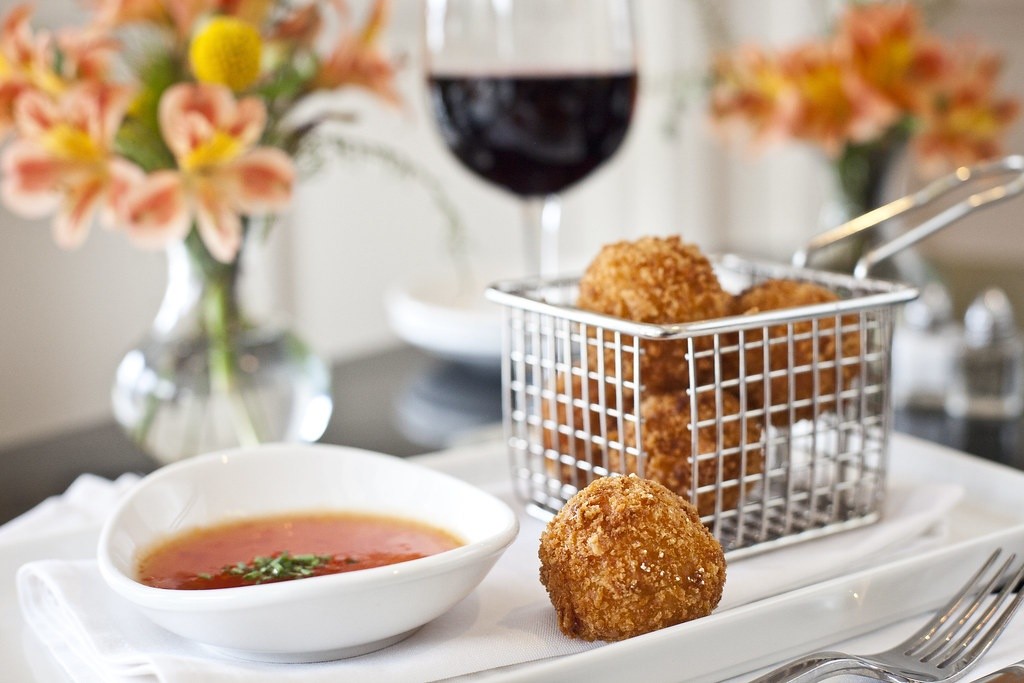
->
[95, 443, 520, 664]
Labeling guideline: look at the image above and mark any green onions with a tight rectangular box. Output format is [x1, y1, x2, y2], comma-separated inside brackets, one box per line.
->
[201, 550, 335, 581]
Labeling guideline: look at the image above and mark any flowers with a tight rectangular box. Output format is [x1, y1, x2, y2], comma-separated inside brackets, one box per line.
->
[712, 9, 1008, 193]
[0, 1, 420, 266]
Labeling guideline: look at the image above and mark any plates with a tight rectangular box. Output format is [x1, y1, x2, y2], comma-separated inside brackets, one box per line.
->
[16, 433, 1023, 682]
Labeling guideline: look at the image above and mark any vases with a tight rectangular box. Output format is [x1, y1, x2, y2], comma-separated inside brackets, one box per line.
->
[107, 202, 335, 465]
[782, 115, 958, 451]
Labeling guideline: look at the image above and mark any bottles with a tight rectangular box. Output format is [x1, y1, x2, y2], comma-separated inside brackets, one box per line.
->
[960, 286, 1024, 415]
[899, 282, 959, 411]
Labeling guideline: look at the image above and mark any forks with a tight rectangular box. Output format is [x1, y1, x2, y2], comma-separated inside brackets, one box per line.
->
[747, 548, 1024, 682]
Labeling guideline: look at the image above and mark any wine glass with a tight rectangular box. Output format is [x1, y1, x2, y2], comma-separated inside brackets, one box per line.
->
[423, 1, 638, 281]
